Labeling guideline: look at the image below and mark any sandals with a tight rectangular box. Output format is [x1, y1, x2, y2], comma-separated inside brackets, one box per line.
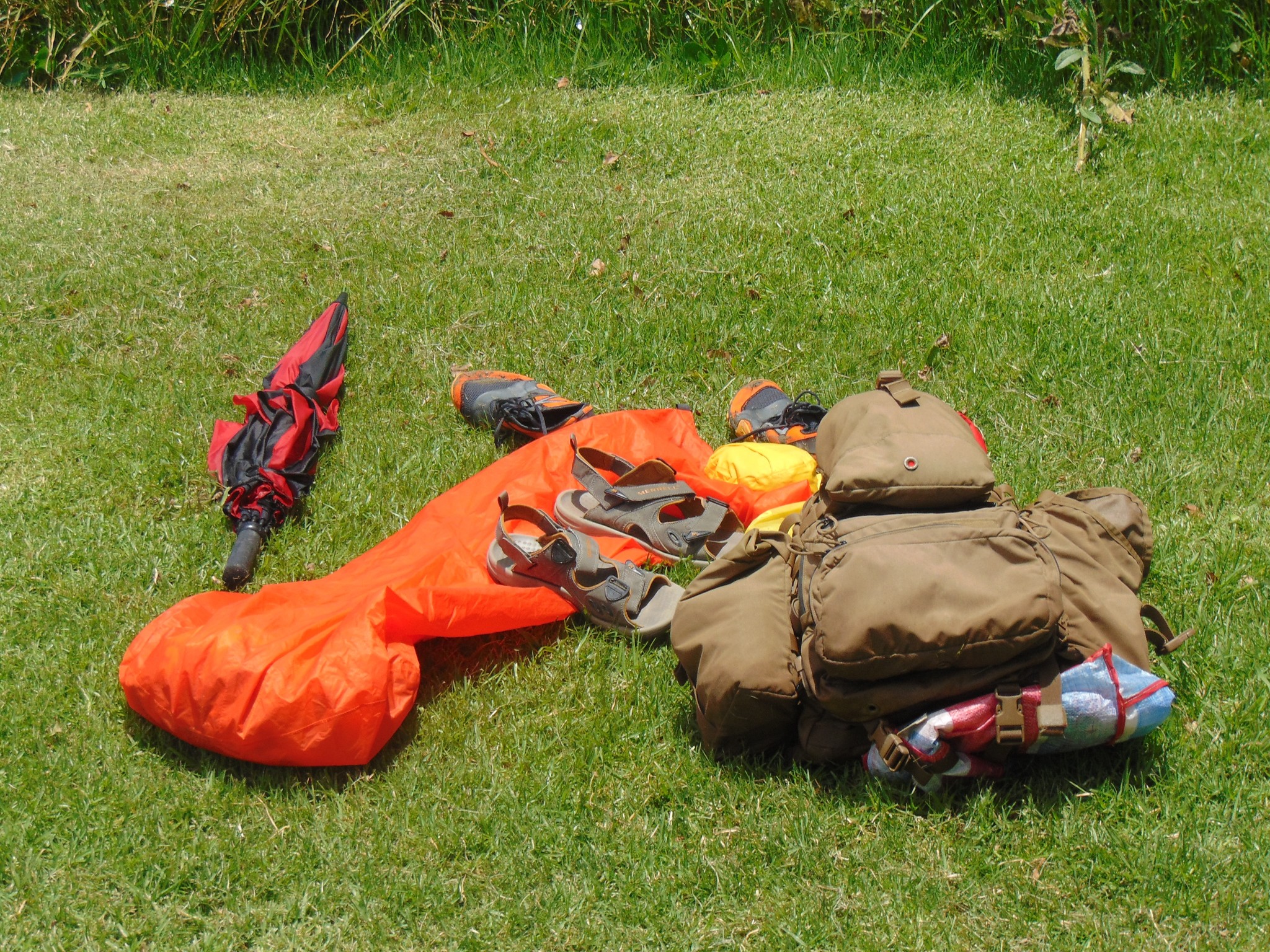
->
[486, 490, 687, 639]
[553, 434, 747, 568]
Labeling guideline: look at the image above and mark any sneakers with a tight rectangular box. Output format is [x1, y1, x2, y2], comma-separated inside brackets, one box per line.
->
[726, 378, 831, 471]
[449, 369, 596, 442]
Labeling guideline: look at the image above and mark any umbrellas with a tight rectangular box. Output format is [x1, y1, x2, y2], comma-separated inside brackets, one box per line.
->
[208, 292, 349, 585]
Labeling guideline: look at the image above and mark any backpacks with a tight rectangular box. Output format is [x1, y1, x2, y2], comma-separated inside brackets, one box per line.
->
[668, 370, 1155, 775]
